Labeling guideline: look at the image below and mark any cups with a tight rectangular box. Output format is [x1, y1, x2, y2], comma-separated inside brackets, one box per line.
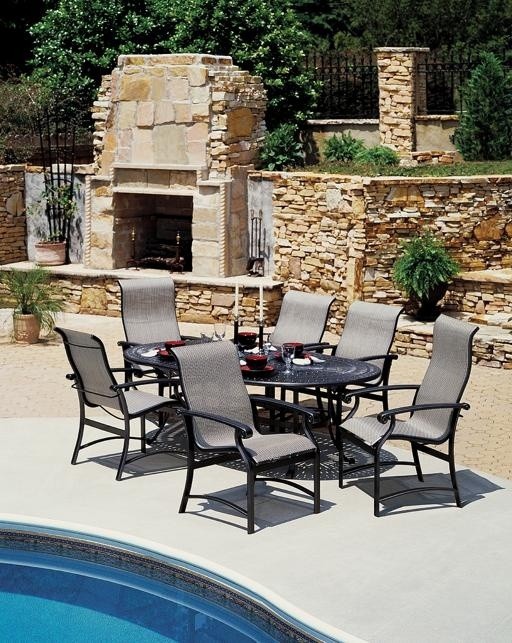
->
[214, 321, 226, 340]
[201, 332, 214, 343]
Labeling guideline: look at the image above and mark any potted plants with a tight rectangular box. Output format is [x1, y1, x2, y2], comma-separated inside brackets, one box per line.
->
[24, 168, 81, 265]
[391, 221, 460, 322]
[0, 263, 68, 344]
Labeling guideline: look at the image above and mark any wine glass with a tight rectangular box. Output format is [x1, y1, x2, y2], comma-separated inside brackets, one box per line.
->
[281, 344, 294, 373]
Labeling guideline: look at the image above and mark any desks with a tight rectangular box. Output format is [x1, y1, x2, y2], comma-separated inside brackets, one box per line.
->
[124, 339, 382, 479]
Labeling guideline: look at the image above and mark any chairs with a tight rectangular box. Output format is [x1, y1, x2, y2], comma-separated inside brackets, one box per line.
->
[300, 301, 405, 429]
[53, 328, 189, 482]
[167, 340, 321, 535]
[241, 291, 336, 410]
[116, 278, 204, 427]
[337, 314, 479, 519]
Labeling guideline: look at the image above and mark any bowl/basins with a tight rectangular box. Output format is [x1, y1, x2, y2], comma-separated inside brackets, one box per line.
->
[245, 354, 266, 370]
[238, 331, 256, 344]
[165, 340, 186, 354]
[283, 343, 304, 353]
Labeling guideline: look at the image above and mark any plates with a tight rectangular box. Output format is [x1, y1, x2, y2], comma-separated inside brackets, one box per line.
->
[236, 342, 258, 348]
[275, 351, 310, 360]
[241, 364, 274, 374]
[157, 350, 175, 358]
